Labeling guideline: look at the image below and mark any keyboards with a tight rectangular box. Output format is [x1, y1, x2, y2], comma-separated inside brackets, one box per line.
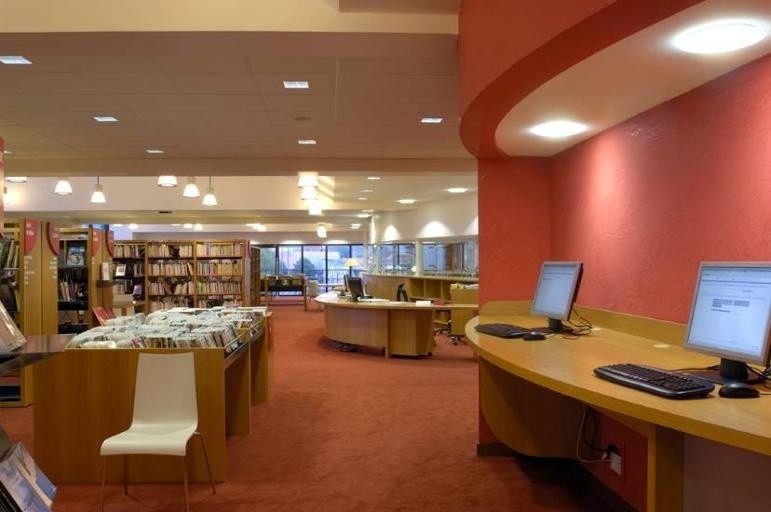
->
[595, 363, 715, 396]
[474, 322, 532, 339]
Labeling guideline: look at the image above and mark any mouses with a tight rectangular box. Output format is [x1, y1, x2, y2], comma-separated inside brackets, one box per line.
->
[721, 380, 759, 399]
[523, 332, 544, 340]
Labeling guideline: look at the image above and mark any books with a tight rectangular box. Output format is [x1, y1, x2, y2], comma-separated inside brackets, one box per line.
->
[0, 235, 269, 375]
[0, 440, 58, 511]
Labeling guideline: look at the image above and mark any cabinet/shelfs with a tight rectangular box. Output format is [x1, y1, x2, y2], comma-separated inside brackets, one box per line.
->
[407, 276, 479, 339]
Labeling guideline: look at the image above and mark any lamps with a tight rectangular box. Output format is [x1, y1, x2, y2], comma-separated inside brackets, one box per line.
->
[53, 173, 327, 238]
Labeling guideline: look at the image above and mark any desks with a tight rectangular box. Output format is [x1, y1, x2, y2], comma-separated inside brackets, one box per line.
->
[313, 291, 435, 359]
[464, 300, 771, 512]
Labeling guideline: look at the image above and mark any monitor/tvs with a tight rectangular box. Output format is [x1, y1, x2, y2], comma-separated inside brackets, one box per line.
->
[682, 260, 771, 383]
[531, 260, 584, 332]
[344, 274, 364, 302]
